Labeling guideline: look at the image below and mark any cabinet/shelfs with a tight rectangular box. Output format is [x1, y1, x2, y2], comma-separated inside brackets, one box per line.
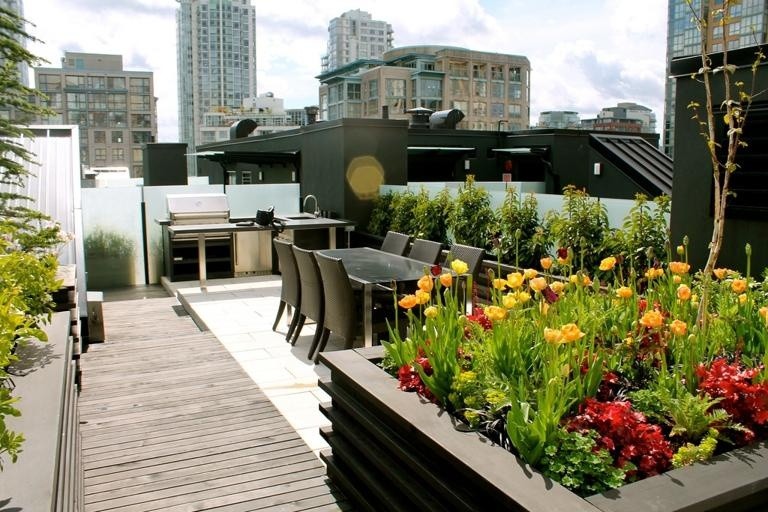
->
[154, 217, 235, 282]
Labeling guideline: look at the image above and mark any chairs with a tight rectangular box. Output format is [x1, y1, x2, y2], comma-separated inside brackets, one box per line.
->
[272, 229, 485, 369]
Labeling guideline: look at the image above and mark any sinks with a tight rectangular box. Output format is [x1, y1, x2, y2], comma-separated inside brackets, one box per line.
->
[286, 216, 316, 220]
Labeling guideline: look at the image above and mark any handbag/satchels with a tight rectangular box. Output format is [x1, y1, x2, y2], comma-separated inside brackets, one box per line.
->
[255, 206, 285, 233]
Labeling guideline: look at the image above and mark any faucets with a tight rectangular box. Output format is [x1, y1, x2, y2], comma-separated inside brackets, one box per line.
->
[303, 194, 321, 218]
